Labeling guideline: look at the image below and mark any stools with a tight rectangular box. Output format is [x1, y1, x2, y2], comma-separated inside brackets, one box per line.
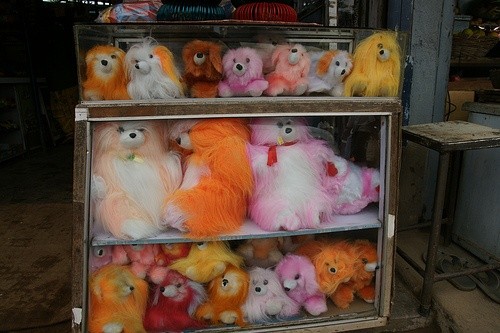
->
[394, 118, 497, 317]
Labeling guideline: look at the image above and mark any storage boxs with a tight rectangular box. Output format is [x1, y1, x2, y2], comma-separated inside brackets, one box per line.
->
[445, 78, 493, 121]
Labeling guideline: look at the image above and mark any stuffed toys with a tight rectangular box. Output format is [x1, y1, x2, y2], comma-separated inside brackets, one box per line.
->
[343, 30, 405, 98]
[122, 37, 190, 99]
[196, 269, 250, 329]
[90, 120, 184, 240]
[329, 239, 379, 310]
[247, 117, 381, 231]
[263, 42, 311, 96]
[274, 254, 330, 316]
[181, 39, 224, 98]
[306, 48, 353, 96]
[219, 45, 270, 96]
[245, 265, 302, 323]
[88, 234, 316, 282]
[293, 236, 353, 296]
[81, 43, 129, 100]
[143, 271, 208, 333]
[160, 117, 252, 239]
[86, 265, 148, 333]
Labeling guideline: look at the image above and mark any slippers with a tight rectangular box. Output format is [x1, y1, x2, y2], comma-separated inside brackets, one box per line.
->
[467, 271, 500, 302]
[423, 248, 476, 291]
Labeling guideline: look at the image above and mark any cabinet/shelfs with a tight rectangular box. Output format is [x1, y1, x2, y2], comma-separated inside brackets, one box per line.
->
[72, 18, 402, 333]
